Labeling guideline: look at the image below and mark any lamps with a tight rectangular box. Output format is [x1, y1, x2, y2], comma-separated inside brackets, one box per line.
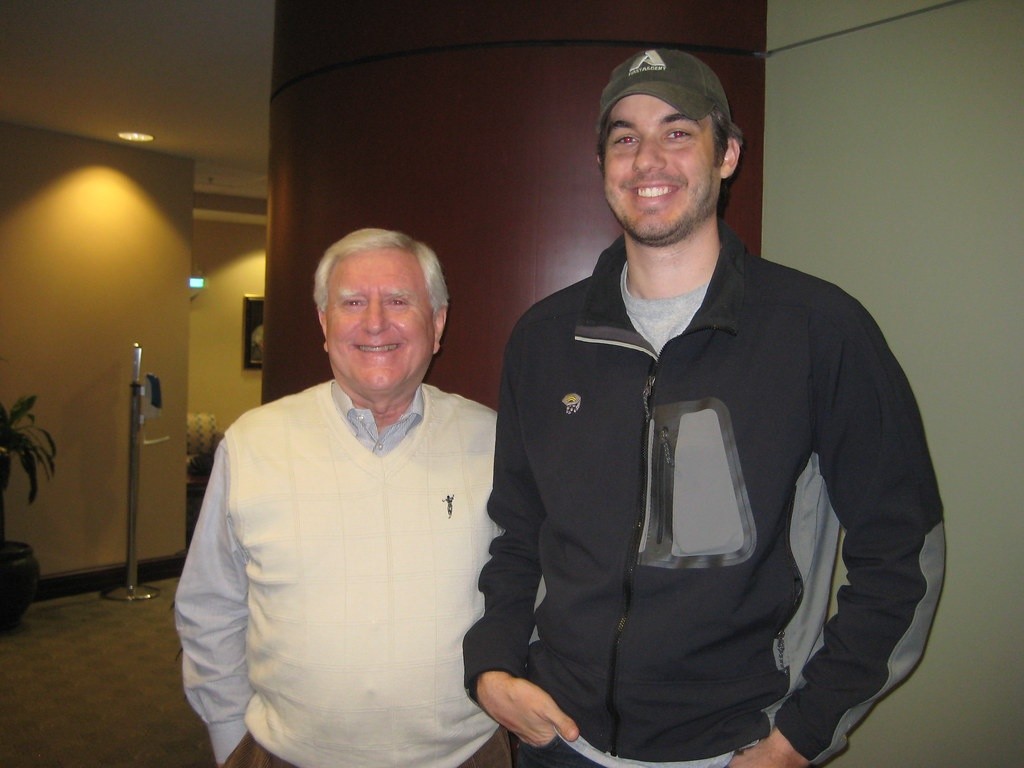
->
[190, 267, 209, 291]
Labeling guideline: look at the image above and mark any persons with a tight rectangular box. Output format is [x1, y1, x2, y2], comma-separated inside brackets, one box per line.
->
[174, 227, 501, 768]
[463, 47, 946, 768]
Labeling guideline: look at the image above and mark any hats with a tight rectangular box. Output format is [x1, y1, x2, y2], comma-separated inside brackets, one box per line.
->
[595, 48, 731, 135]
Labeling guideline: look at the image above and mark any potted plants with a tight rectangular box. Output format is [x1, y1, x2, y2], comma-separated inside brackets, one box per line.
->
[0, 391, 59, 636]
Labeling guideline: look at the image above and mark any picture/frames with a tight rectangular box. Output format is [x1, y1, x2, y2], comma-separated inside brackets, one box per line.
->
[241, 294, 263, 370]
